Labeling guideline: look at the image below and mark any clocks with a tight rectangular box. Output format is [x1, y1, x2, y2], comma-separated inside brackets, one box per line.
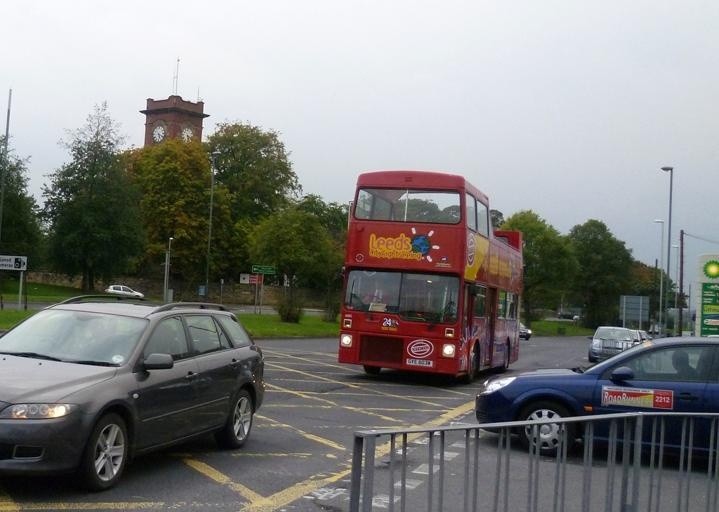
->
[151, 123, 195, 146]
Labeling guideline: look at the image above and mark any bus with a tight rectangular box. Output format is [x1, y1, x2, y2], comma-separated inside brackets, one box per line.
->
[338, 171, 523, 382]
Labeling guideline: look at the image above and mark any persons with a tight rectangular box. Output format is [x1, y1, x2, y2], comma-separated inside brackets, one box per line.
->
[672, 350, 696, 377]
[364, 277, 393, 304]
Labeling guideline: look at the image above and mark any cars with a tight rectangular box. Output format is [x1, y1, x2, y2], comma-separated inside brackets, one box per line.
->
[556, 312, 584, 320]
[104, 284, 144, 298]
[519, 322, 532, 340]
[0, 295, 264, 489]
[477, 325, 719, 457]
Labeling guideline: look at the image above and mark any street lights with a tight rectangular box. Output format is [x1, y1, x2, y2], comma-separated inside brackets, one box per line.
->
[662, 166, 674, 336]
[166, 237, 174, 303]
[205, 150, 222, 300]
[653, 220, 665, 335]
